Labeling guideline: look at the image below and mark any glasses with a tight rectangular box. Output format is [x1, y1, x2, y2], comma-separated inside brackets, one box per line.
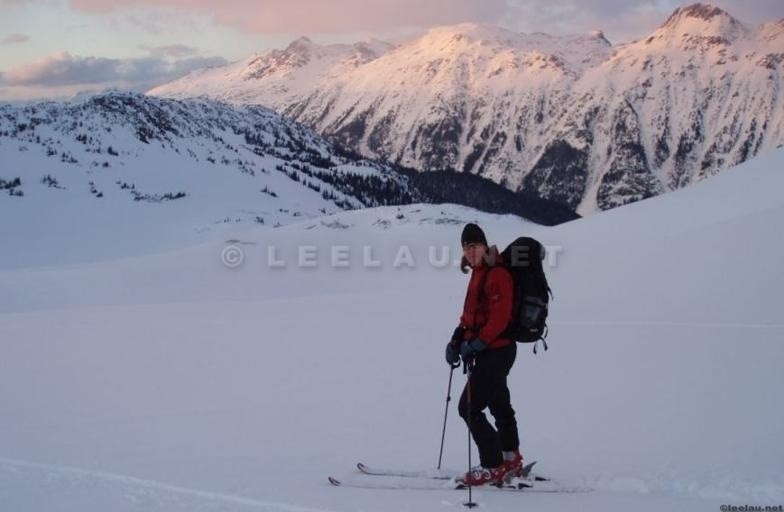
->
[464, 242, 481, 250]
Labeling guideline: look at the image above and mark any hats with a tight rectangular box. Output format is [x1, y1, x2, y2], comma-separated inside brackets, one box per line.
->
[461, 224, 487, 245]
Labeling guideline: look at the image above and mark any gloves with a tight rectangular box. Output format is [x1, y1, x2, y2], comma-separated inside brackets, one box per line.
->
[445, 328, 463, 368]
[462, 338, 487, 361]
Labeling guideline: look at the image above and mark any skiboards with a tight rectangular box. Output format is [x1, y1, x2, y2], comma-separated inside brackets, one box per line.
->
[328, 463, 552, 489]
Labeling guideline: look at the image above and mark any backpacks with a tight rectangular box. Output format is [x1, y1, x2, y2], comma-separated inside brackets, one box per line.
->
[478, 237, 548, 343]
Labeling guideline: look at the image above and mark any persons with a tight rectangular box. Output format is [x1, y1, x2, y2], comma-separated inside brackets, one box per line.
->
[446, 223, 519, 486]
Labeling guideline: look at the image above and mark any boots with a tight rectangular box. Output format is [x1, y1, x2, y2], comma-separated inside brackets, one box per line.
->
[501, 448, 525, 471]
[455, 466, 506, 485]
